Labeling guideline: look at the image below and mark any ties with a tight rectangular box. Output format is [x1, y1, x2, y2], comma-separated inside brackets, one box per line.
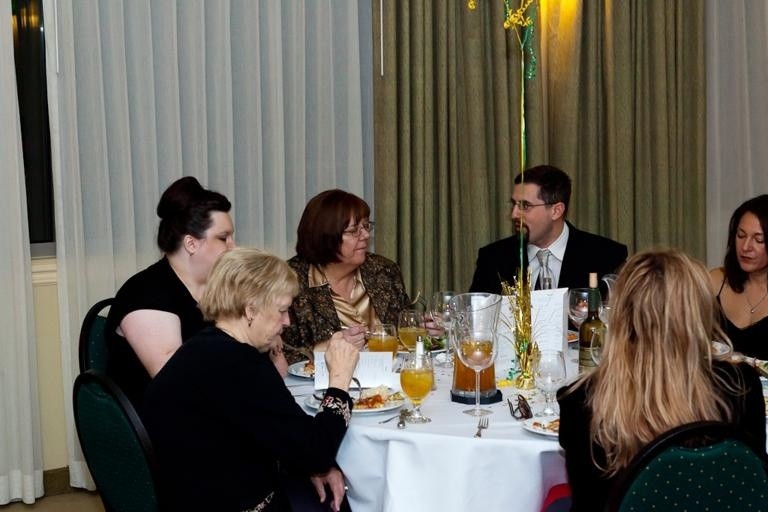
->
[535, 249, 556, 291]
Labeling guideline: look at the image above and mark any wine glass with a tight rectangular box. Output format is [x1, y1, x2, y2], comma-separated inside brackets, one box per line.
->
[399, 355, 434, 424]
[533, 349, 567, 417]
[398, 310, 426, 367]
[457, 327, 498, 416]
[569, 288, 603, 364]
[429, 295, 464, 369]
[368, 324, 398, 362]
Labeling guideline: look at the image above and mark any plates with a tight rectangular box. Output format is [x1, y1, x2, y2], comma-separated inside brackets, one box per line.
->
[305, 389, 404, 416]
[704, 340, 731, 360]
[288, 359, 315, 379]
[522, 416, 559, 441]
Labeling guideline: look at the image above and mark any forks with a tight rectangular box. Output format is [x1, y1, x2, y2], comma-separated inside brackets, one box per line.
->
[281, 342, 313, 363]
[473, 417, 489, 438]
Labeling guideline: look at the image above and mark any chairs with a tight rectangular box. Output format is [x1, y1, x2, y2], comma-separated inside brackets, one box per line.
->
[73, 369, 164, 512]
[609, 419, 768, 512]
[78, 297, 115, 374]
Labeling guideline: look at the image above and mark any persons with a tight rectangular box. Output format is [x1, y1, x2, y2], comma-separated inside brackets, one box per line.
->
[146, 247, 361, 512]
[706, 193, 768, 375]
[280, 189, 446, 366]
[555, 247, 768, 512]
[104, 176, 235, 409]
[469, 164, 628, 331]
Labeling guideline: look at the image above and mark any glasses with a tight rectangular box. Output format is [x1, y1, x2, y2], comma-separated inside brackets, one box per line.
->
[506, 201, 558, 211]
[344, 221, 376, 237]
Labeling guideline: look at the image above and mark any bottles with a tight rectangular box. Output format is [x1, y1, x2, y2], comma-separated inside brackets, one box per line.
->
[415, 335, 424, 369]
[578, 273, 607, 378]
[424, 351, 437, 390]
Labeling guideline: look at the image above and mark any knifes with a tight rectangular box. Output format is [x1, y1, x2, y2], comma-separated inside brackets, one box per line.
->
[397, 408, 408, 429]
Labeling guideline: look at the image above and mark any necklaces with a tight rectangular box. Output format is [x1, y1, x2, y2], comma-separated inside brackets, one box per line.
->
[743, 284, 768, 313]
